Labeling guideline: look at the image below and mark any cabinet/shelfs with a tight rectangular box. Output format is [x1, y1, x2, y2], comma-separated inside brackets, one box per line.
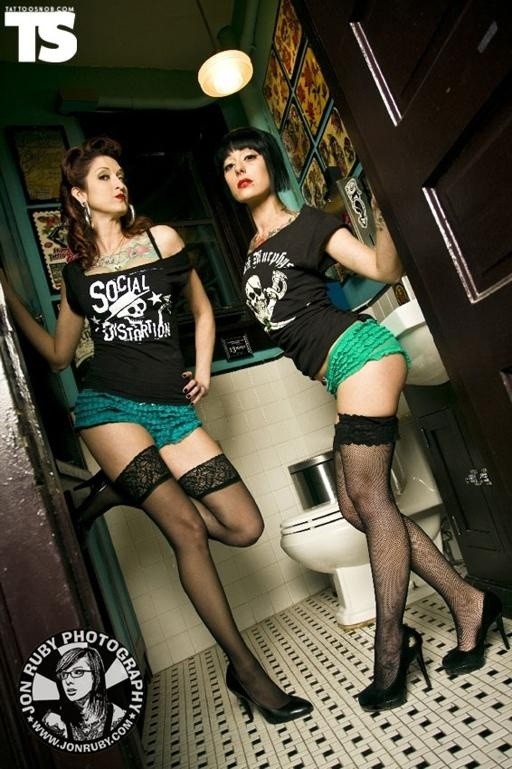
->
[391, 363, 511, 620]
[2, 291, 157, 762]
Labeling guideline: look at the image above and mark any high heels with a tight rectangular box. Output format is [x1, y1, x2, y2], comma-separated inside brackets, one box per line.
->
[70, 466, 112, 547]
[224, 663, 314, 725]
[357, 622, 433, 713]
[440, 588, 510, 676]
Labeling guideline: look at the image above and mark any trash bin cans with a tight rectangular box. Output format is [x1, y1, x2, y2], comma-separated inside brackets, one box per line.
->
[288, 451, 337, 511]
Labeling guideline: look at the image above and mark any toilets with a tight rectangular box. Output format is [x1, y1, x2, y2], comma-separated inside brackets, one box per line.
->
[277, 299, 443, 627]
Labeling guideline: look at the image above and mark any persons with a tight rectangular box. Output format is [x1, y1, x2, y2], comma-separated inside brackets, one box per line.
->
[1, 136, 315, 726]
[217, 121, 510, 717]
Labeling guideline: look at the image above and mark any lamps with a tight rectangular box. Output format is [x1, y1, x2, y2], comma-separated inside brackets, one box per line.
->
[183, 0, 256, 98]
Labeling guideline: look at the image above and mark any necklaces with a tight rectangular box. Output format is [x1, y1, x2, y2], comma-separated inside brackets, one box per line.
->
[100, 232, 131, 274]
[250, 214, 297, 246]
